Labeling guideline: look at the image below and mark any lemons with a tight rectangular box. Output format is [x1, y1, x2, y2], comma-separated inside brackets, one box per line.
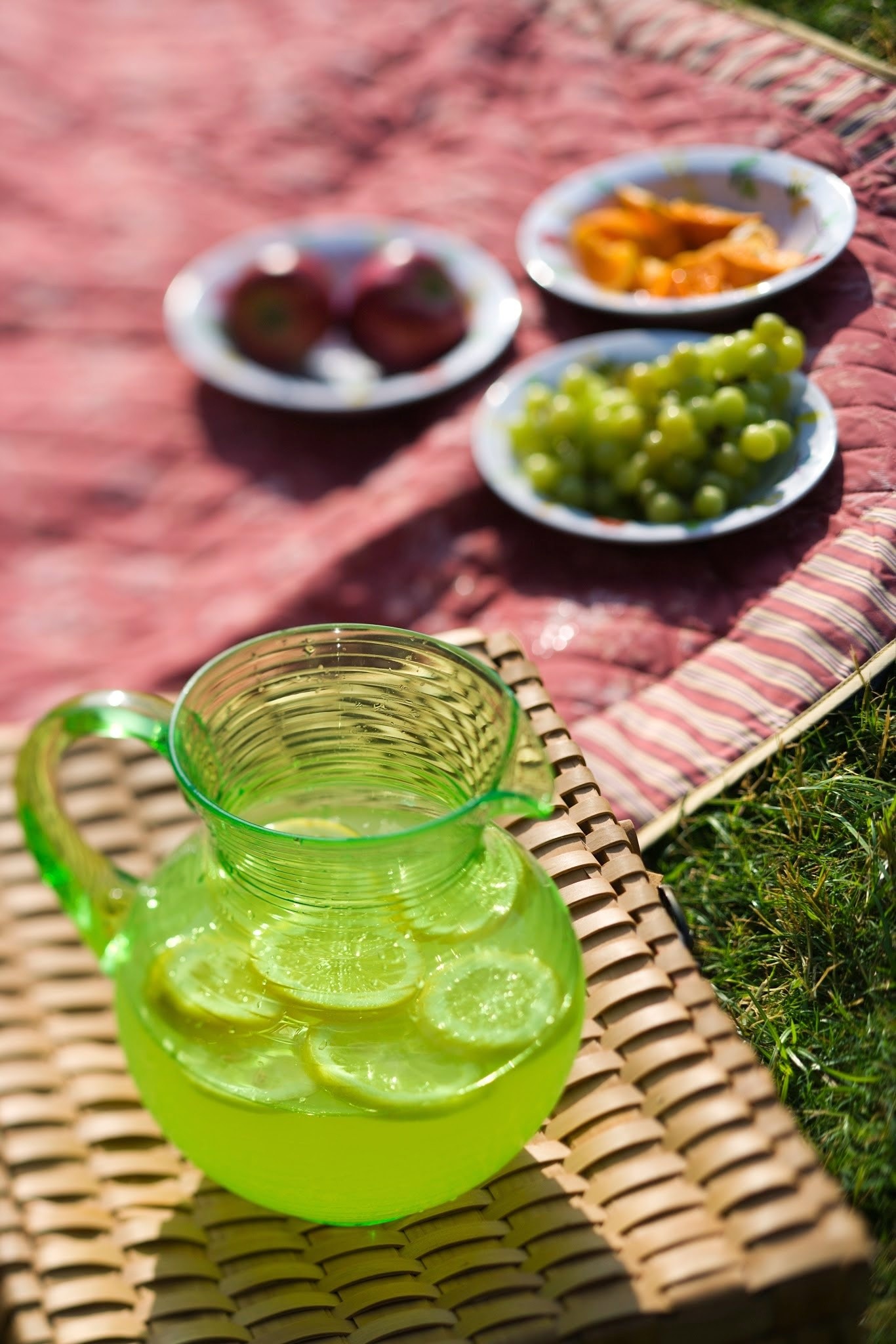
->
[152, 818, 561, 1128]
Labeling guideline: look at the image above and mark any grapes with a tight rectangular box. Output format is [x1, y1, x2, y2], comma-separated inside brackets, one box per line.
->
[508, 313, 807, 519]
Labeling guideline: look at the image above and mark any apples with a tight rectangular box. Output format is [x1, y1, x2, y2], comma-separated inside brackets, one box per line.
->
[224, 238, 466, 376]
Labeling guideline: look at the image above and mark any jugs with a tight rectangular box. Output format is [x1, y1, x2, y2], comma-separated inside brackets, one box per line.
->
[14, 623, 582, 1226]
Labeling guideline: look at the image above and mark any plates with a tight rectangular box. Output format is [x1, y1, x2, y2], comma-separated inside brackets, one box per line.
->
[162, 214, 523, 413]
[471, 327, 836, 541]
[515, 145, 857, 315]
